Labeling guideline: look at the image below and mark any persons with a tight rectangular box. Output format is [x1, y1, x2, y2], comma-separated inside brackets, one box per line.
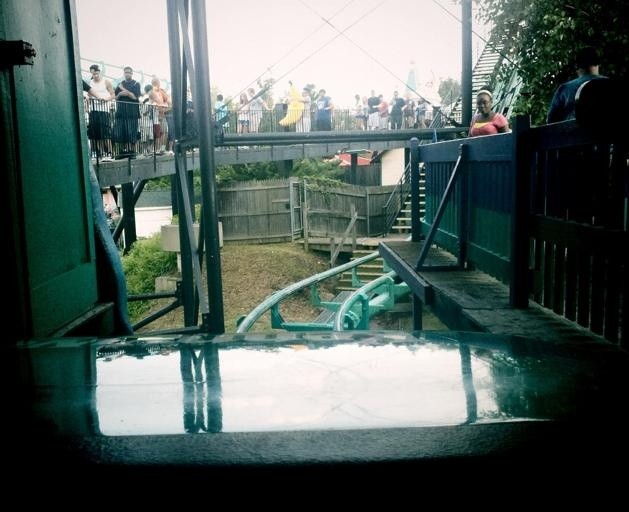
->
[354, 89, 428, 130]
[82, 65, 176, 159]
[466, 85, 509, 137]
[238, 78, 332, 134]
[546, 46, 609, 124]
[186, 91, 239, 134]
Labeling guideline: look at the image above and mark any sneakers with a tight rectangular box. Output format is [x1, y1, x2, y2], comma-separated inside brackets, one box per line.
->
[114, 151, 137, 161]
